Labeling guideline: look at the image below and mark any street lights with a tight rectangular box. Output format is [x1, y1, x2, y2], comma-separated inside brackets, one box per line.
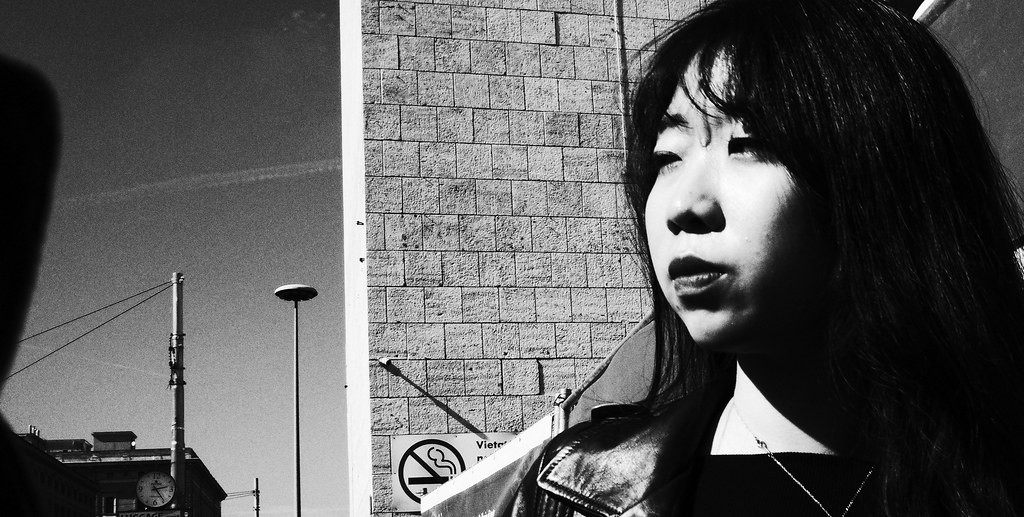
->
[273, 284, 319, 514]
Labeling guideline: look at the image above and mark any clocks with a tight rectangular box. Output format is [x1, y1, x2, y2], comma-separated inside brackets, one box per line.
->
[135, 468, 177, 510]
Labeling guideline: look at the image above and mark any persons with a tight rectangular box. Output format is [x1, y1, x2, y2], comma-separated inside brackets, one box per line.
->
[491, 0, 1024, 517]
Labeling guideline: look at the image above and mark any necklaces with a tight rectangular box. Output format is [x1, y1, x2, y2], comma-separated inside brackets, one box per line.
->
[724, 384, 875, 517]
